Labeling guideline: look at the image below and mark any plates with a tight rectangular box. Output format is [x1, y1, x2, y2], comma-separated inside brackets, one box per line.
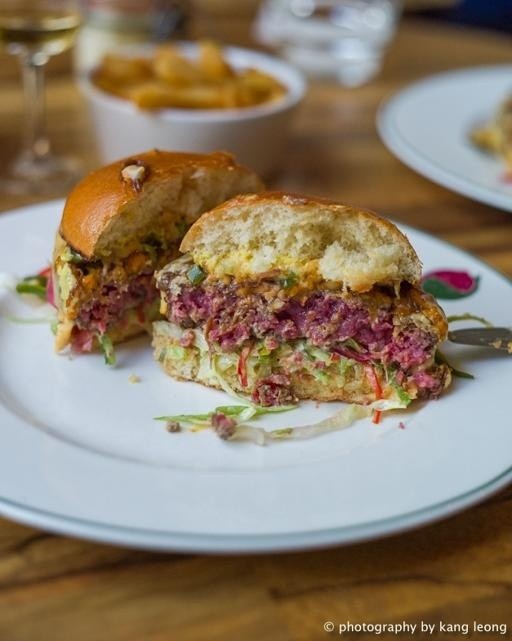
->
[378, 66, 512, 216]
[0, 193, 512, 558]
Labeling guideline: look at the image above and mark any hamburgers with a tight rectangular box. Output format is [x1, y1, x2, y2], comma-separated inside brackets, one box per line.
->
[151, 190, 453, 406]
[46, 151, 267, 354]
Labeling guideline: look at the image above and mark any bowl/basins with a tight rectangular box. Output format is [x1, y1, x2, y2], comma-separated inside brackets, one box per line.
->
[77, 44, 314, 182]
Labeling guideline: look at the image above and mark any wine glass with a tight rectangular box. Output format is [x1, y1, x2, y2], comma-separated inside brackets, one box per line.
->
[2, 1, 93, 191]
